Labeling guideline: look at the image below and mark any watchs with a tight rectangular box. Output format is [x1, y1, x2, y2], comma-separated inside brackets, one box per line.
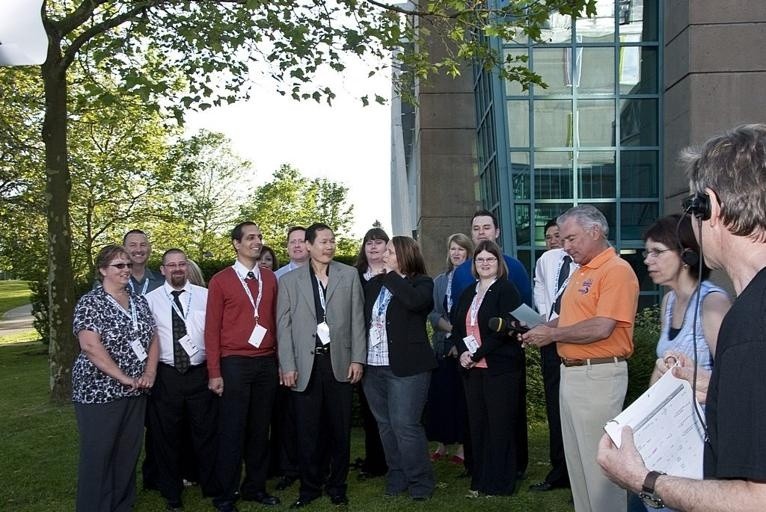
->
[638, 469, 668, 509]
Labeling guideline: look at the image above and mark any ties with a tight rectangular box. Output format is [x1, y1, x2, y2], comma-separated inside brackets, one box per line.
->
[169, 290, 191, 375]
[248, 271, 254, 280]
[555, 255, 573, 316]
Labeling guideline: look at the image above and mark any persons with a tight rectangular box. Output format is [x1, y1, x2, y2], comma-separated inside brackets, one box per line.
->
[427, 230, 474, 467]
[351, 226, 390, 483]
[276, 222, 369, 507]
[528, 244, 578, 494]
[594, 119, 766, 511]
[642, 212, 735, 417]
[203, 220, 283, 512]
[449, 210, 535, 481]
[451, 238, 525, 500]
[142, 248, 225, 510]
[359, 233, 442, 502]
[254, 244, 275, 269]
[516, 203, 642, 511]
[542, 217, 561, 250]
[187, 257, 207, 288]
[273, 226, 312, 491]
[92, 228, 166, 491]
[68, 244, 159, 512]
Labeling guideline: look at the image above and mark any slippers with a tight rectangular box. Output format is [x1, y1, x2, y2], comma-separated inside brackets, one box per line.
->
[429, 448, 449, 463]
[450, 451, 464, 465]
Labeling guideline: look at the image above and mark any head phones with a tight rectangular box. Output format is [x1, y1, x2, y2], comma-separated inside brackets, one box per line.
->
[690, 158, 712, 221]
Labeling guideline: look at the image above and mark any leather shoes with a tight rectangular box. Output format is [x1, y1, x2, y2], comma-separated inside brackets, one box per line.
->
[213, 498, 238, 512]
[357, 455, 387, 482]
[288, 491, 322, 509]
[240, 487, 280, 505]
[325, 486, 348, 506]
[276, 471, 300, 490]
[529, 478, 571, 493]
[164, 493, 185, 512]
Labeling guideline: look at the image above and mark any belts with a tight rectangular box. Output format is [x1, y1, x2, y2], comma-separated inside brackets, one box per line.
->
[314, 346, 330, 355]
[223, 354, 275, 360]
[560, 354, 626, 368]
[159, 360, 208, 370]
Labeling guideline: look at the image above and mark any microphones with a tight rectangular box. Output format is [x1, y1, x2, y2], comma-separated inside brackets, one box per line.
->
[487, 316, 529, 336]
[672, 212, 699, 268]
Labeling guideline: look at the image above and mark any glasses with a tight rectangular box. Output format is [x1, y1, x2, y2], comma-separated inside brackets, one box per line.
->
[642, 247, 686, 257]
[102, 263, 133, 269]
